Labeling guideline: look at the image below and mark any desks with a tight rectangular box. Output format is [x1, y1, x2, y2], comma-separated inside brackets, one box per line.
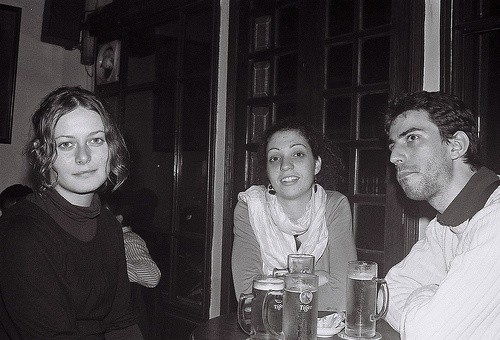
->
[187, 308, 402, 340]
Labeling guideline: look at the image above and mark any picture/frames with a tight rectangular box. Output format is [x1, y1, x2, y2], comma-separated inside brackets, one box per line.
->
[0, 2, 22, 144]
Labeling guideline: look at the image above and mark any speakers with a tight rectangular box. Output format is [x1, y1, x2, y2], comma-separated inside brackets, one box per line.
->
[40, 0, 84, 48]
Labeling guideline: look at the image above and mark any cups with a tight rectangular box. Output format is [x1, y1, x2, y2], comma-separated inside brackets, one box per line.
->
[272, 253, 315, 276]
[344, 261, 389, 338]
[262, 272, 317, 340]
[237, 277, 284, 340]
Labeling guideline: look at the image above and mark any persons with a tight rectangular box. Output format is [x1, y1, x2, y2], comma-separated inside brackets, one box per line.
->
[377, 90, 500, 340]
[0, 87, 143, 340]
[231, 119, 357, 311]
[102, 192, 161, 288]
[0, 184, 34, 217]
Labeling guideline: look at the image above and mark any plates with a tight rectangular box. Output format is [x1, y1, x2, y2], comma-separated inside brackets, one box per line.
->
[317, 317, 345, 337]
